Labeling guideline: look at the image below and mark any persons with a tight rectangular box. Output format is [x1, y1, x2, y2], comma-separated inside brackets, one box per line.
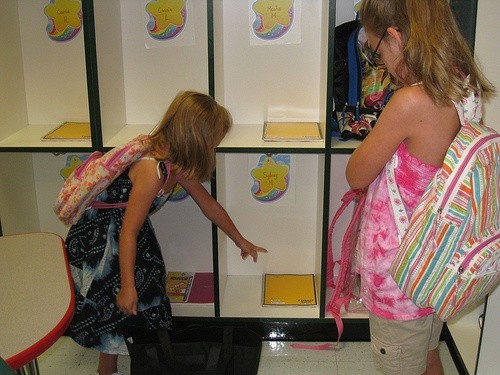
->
[55, 89, 268, 375]
[345, 0, 496, 375]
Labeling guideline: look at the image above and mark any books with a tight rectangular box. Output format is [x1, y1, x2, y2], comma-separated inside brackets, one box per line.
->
[42, 121, 92, 142]
[262, 120, 323, 142]
[166, 270, 214, 303]
[264, 273, 318, 306]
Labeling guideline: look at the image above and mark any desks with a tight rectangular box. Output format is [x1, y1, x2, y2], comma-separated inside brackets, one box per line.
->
[0, 228, 71, 375]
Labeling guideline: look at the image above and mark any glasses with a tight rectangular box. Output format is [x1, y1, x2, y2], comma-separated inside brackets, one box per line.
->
[363, 27, 401, 67]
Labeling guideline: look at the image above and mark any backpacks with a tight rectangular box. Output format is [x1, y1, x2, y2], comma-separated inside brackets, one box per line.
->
[380, 82, 500, 322]
[55, 134, 175, 225]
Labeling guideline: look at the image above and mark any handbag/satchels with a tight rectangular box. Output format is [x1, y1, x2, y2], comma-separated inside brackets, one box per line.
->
[121, 312, 263, 375]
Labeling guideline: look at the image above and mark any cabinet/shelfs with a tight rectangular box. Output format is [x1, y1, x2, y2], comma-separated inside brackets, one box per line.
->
[0, 0, 480, 345]
